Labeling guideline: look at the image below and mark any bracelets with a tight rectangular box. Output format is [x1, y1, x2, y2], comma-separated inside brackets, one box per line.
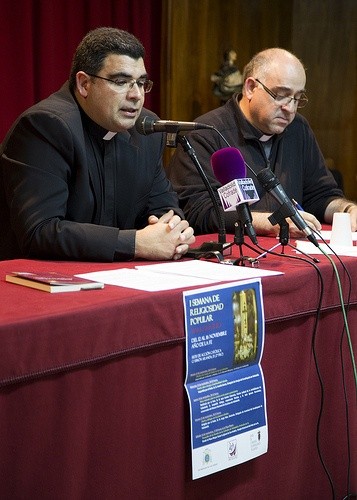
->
[343, 203, 355, 213]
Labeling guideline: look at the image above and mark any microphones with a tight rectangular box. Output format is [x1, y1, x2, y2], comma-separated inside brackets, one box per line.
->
[135, 114, 215, 135]
[256, 168, 320, 247]
[211, 147, 260, 245]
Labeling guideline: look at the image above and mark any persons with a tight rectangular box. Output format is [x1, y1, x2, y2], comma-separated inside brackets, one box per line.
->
[165, 48, 357, 238]
[0, 27, 196, 261]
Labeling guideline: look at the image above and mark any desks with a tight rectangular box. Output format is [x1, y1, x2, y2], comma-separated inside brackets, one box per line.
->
[1, 224, 356, 500]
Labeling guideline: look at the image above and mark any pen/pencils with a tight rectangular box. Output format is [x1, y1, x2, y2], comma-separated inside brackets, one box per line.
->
[292, 198, 323, 236]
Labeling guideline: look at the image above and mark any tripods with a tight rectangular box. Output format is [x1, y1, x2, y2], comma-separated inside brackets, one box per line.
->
[206, 219, 266, 266]
[255, 202, 321, 263]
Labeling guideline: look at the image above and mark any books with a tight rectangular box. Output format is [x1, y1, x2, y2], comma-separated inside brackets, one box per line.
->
[7, 272, 104, 294]
